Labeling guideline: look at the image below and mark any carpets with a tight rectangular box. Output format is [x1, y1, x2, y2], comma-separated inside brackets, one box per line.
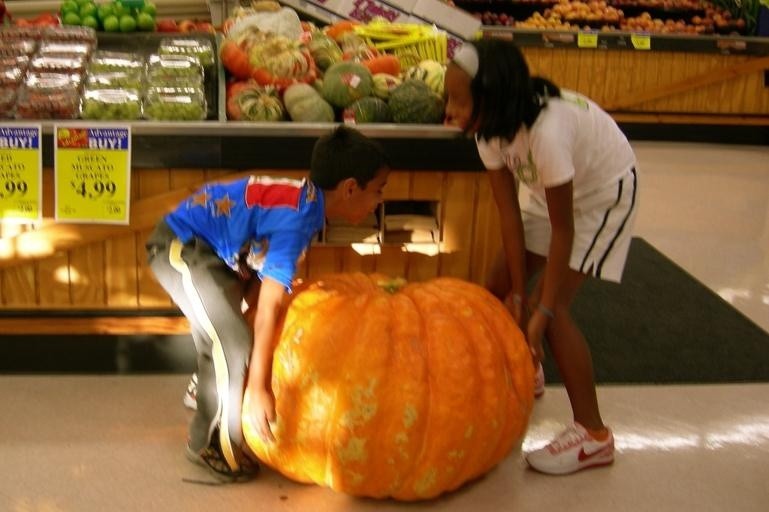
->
[2, 233, 769, 385]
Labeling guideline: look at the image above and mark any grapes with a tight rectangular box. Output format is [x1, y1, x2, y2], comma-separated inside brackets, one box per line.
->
[80, 38, 214, 121]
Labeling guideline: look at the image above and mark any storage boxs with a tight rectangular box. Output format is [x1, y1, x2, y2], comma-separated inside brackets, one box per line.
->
[281, 0, 481, 67]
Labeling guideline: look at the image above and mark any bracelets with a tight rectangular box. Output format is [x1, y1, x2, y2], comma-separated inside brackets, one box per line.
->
[537, 302, 555, 319]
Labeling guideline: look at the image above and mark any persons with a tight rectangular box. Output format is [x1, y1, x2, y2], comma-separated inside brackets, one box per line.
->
[445, 38, 640, 475]
[146, 125, 392, 482]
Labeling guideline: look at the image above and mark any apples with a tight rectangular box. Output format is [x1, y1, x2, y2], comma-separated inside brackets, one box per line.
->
[61, 0, 216, 34]
[482, 0, 732, 34]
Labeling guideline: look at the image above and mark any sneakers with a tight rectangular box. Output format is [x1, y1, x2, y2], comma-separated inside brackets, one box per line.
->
[525, 422, 615, 475]
[185, 434, 260, 484]
[183, 372, 198, 411]
[531, 361, 545, 398]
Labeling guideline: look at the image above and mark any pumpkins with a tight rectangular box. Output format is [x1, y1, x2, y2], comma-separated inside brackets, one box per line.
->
[241, 272, 536, 503]
[220, 21, 446, 123]
